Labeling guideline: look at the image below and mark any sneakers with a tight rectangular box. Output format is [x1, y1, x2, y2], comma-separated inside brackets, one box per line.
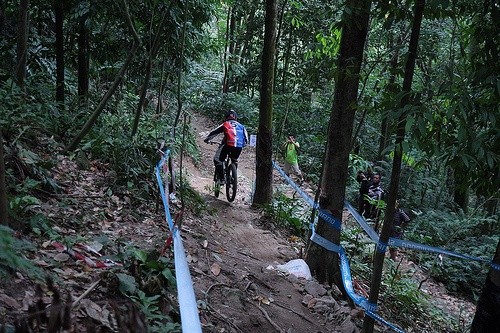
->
[217, 179, 225, 186]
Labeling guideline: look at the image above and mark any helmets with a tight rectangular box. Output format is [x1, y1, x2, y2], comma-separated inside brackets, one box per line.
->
[223, 110, 237, 122]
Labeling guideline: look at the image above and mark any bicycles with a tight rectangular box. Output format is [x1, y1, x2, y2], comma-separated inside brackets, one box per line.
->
[205, 140, 238, 203]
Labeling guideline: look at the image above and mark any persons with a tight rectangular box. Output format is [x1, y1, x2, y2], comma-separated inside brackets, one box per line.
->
[357, 162, 374, 217]
[204, 109, 248, 186]
[365, 172, 385, 220]
[282, 134, 304, 183]
[389, 199, 410, 264]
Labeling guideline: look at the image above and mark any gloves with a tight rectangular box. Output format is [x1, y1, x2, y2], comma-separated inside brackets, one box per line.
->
[203, 139, 210, 142]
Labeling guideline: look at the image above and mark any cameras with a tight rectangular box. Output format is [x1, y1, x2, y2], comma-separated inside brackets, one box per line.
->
[289, 137, 291, 140]
